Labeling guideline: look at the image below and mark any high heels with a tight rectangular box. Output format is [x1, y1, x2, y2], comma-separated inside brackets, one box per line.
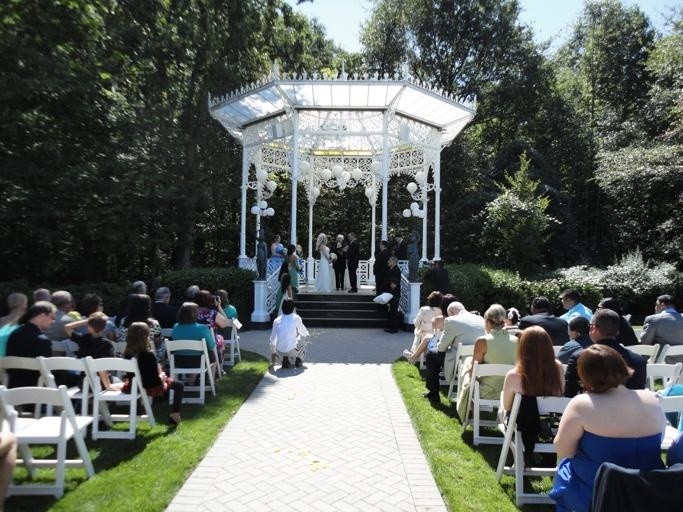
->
[295, 357, 303, 368]
[282, 355, 292, 368]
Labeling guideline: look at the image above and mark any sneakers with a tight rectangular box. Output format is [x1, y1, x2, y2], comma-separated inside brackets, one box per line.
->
[403, 349, 416, 365]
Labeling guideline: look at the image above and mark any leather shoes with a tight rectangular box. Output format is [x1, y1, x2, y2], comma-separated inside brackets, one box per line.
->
[420, 394, 440, 402]
[169, 412, 182, 425]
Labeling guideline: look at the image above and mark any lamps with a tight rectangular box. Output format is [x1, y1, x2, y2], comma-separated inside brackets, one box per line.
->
[298, 161, 321, 206]
[363, 157, 384, 210]
[248, 165, 280, 219]
[319, 164, 361, 190]
[400, 168, 434, 219]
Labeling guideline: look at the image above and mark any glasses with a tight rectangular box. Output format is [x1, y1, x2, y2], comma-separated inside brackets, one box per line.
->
[49, 316, 56, 323]
[588, 323, 594, 329]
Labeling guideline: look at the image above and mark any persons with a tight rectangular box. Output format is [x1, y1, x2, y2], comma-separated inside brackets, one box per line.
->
[550, 342, 667, 510]
[268, 232, 407, 334]
[1, 281, 236, 424]
[268, 298, 309, 368]
[0, 429, 18, 509]
[495, 326, 561, 476]
[424, 260, 449, 295]
[405, 290, 683, 423]
